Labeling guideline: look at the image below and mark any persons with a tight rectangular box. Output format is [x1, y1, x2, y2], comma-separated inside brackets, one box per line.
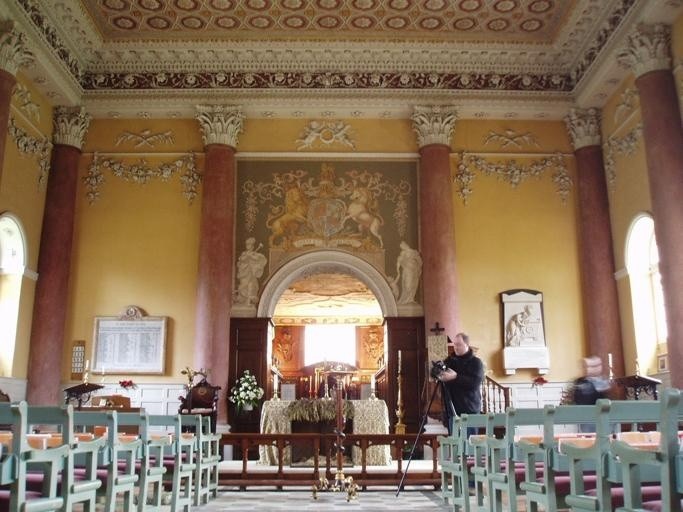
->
[385, 270, 402, 298]
[507, 312, 528, 346]
[572, 355, 615, 432]
[436, 332, 485, 441]
[237, 236, 268, 306]
[397, 240, 422, 304]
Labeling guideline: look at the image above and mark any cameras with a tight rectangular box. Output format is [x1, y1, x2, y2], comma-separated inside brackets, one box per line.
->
[430, 359, 447, 378]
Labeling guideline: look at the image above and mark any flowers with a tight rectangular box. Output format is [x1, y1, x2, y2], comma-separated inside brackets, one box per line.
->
[227, 369, 264, 417]
[531, 378, 547, 388]
[118, 380, 132, 389]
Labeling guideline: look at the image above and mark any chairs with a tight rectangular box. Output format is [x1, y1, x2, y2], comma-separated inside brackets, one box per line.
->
[177, 379, 221, 436]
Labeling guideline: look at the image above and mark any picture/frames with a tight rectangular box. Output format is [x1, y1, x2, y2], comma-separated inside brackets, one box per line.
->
[656, 353, 668, 372]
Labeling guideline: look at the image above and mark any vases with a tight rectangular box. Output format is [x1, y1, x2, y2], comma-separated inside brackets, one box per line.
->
[241, 403, 253, 412]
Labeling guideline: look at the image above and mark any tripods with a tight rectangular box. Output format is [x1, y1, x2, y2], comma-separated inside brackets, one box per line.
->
[395, 378, 461, 497]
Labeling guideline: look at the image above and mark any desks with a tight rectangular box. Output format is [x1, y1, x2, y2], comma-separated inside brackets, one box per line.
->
[259, 400, 389, 468]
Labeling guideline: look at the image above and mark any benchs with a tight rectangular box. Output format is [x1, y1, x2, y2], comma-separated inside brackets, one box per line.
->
[436, 387, 682, 512]
[0, 399, 222, 512]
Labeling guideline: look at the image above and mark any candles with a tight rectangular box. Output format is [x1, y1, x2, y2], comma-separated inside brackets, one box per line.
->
[606, 352, 612, 375]
[370, 375, 375, 389]
[397, 350, 401, 373]
[309, 376, 316, 392]
[86, 359, 89, 368]
